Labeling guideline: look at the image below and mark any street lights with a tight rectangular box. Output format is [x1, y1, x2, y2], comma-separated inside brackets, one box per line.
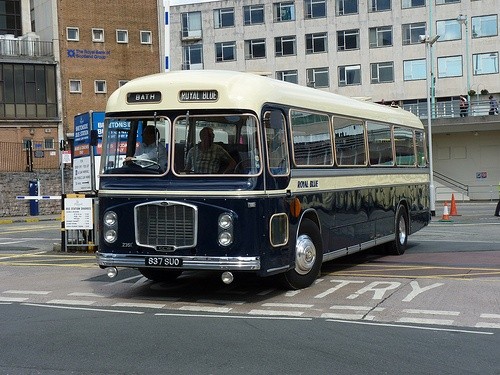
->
[424, 34, 441, 216]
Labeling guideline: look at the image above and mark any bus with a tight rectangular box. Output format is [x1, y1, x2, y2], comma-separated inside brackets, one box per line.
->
[89, 69, 432, 290]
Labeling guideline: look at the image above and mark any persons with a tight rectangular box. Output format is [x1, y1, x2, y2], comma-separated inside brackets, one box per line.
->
[488, 94, 499, 116]
[124, 125, 168, 174]
[459, 95, 467, 118]
[182, 127, 234, 174]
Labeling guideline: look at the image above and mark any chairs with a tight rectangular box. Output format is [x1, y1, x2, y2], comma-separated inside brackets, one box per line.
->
[186, 139, 398, 173]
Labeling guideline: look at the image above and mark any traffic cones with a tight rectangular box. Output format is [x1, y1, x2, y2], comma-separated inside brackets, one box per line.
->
[439, 201, 454, 222]
[448, 194, 462, 216]
